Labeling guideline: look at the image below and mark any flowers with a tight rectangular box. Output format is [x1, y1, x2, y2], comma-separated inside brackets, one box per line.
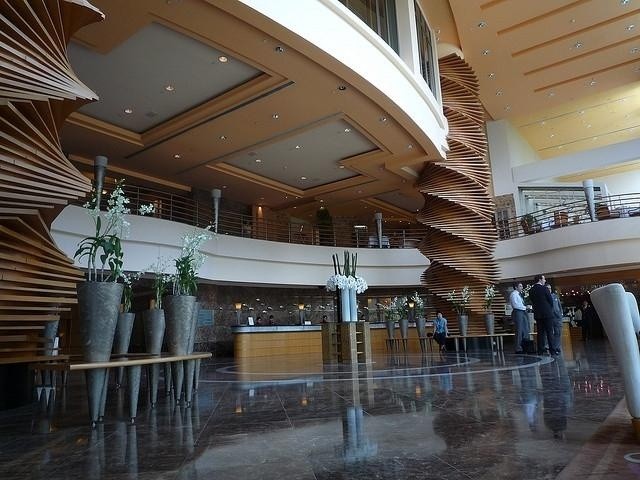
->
[448, 286, 475, 316]
[481, 284, 496, 314]
[520, 285, 533, 312]
[73, 178, 230, 313]
[383, 292, 426, 320]
[325, 250, 368, 294]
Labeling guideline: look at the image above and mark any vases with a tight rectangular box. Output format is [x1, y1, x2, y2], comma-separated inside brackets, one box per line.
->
[337, 288, 358, 323]
[37, 282, 199, 362]
[398, 319, 409, 339]
[417, 318, 426, 337]
[459, 315, 468, 336]
[486, 314, 495, 334]
[386, 320, 395, 338]
[527, 313, 534, 333]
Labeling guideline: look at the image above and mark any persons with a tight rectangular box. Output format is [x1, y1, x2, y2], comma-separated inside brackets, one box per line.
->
[566, 301, 594, 342]
[320, 316, 328, 323]
[430, 312, 448, 351]
[256, 317, 262, 325]
[509, 274, 563, 358]
[269, 315, 274, 325]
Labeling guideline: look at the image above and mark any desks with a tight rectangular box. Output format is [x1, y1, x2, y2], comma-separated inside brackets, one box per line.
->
[27, 352, 212, 429]
[385, 332, 538, 353]
[368, 236, 389, 248]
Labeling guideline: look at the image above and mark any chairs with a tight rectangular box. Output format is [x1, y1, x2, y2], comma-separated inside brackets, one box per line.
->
[520, 204, 619, 234]
